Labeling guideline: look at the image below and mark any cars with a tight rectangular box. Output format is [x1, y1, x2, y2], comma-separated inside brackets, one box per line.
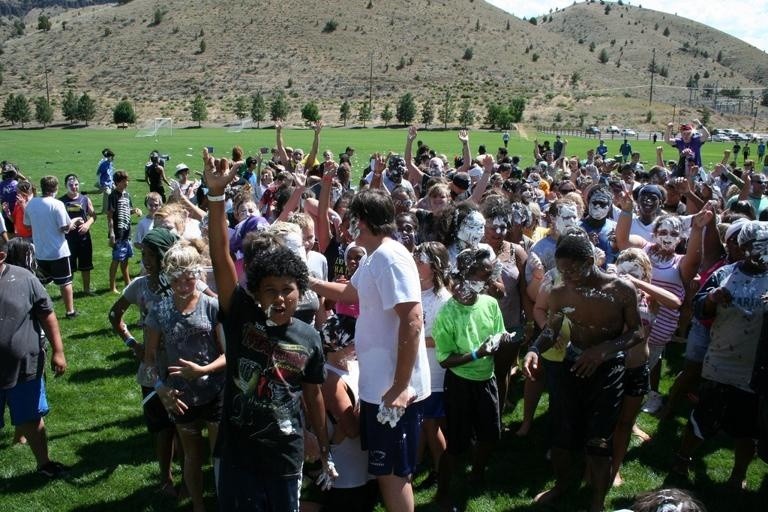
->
[585, 124, 768, 143]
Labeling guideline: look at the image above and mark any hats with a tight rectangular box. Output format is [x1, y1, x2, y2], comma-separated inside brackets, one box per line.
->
[638, 184, 661, 199]
[174, 163, 191, 176]
[680, 123, 694, 131]
[725, 222, 745, 240]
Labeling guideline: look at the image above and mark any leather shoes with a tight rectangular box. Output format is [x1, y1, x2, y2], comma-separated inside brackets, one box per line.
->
[66, 310, 80, 319]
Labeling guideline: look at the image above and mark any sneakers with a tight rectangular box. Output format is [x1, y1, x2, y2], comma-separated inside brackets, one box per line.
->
[37, 461, 71, 479]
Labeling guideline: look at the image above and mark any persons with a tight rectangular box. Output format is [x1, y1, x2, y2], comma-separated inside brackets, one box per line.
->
[1, 118, 768, 512]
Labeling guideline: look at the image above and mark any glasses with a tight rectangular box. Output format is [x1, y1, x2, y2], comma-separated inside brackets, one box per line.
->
[743, 163, 750, 166]
[752, 179, 767, 184]
[560, 188, 576, 195]
